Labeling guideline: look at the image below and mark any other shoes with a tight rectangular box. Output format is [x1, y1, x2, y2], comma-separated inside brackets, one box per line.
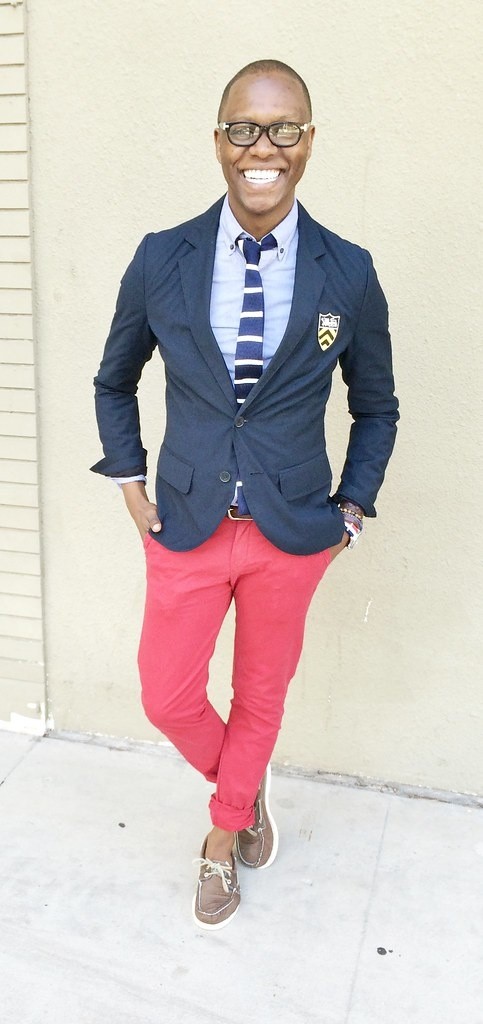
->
[235, 759, 279, 872]
[192, 832, 242, 932]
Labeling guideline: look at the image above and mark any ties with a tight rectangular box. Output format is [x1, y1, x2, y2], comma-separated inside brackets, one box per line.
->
[234, 233, 278, 409]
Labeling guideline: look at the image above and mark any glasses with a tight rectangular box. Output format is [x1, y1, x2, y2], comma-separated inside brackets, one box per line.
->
[218, 121, 311, 148]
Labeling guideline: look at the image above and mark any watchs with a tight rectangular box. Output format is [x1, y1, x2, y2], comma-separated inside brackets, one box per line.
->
[345, 522, 361, 550]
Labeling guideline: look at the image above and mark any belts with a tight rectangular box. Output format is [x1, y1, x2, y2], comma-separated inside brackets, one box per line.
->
[227, 506, 254, 520]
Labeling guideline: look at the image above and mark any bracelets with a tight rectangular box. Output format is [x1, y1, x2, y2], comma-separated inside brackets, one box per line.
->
[336, 500, 363, 533]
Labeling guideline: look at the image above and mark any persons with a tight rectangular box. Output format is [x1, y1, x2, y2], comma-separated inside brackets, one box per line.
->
[90, 59, 400, 928]
